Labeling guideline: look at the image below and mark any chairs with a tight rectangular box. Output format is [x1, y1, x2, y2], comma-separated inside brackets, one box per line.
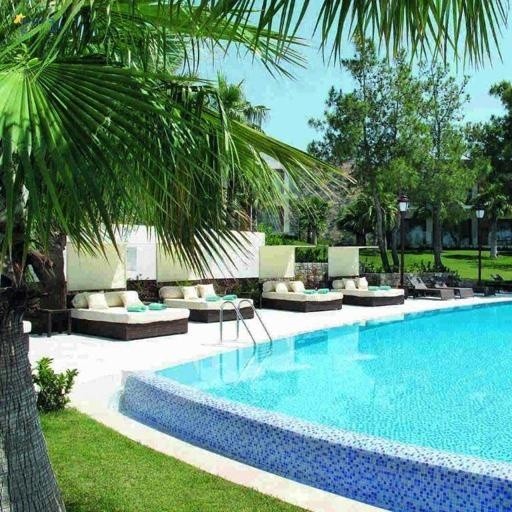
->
[405, 274, 475, 301]
[36, 280, 73, 337]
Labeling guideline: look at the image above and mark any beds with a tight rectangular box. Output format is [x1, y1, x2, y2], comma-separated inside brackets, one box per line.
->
[159, 284, 254, 323]
[69, 291, 191, 341]
[260, 280, 344, 312]
[330, 278, 405, 307]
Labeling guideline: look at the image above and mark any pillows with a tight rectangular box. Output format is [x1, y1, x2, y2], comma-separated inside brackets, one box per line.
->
[289, 281, 304, 292]
[196, 285, 216, 298]
[273, 283, 288, 294]
[181, 286, 198, 300]
[342, 279, 356, 289]
[85, 291, 109, 310]
[356, 278, 368, 289]
[118, 291, 144, 308]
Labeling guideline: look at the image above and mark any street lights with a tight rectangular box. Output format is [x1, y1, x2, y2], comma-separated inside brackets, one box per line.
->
[396, 194, 411, 299]
[473, 199, 487, 294]
